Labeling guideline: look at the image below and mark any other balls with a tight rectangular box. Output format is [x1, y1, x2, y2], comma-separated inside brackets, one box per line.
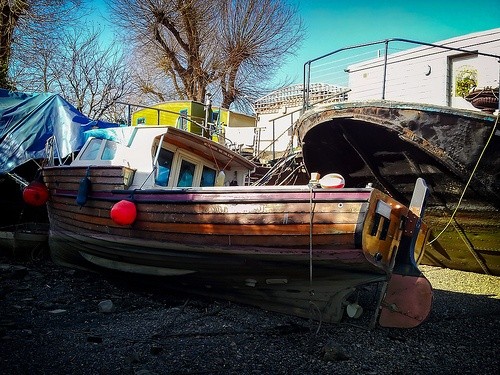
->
[319, 172, 346, 189]
[23, 180, 49, 206]
[109, 199, 137, 226]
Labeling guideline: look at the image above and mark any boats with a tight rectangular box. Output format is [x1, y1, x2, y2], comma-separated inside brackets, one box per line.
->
[289, 37, 499, 276]
[38, 123, 435, 331]
